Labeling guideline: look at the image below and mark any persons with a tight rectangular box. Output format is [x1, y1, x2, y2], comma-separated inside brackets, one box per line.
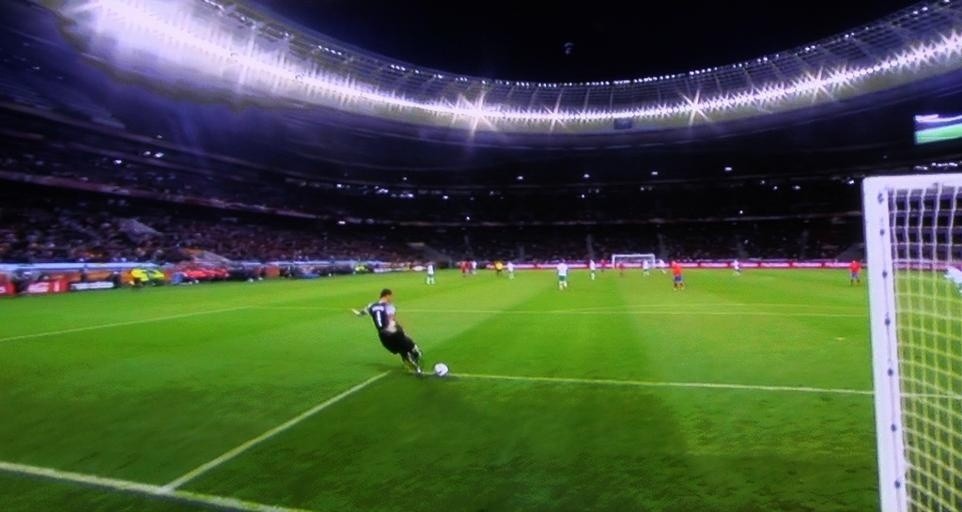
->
[642, 259, 651, 275]
[734, 259, 742, 273]
[556, 258, 568, 288]
[657, 258, 666, 274]
[507, 260, 514, 278]
[0, 148, 866, 296]
[590, 260, 596, 280]
[850, 261, 860, 285]
[671, 261, 685, 291]
[496, 261, 504, 277]
[349, 290, 422, 377]
[426, 264, 435, 285]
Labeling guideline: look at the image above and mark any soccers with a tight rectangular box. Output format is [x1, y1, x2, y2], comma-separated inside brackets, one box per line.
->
[435, 364, 447, 377]
